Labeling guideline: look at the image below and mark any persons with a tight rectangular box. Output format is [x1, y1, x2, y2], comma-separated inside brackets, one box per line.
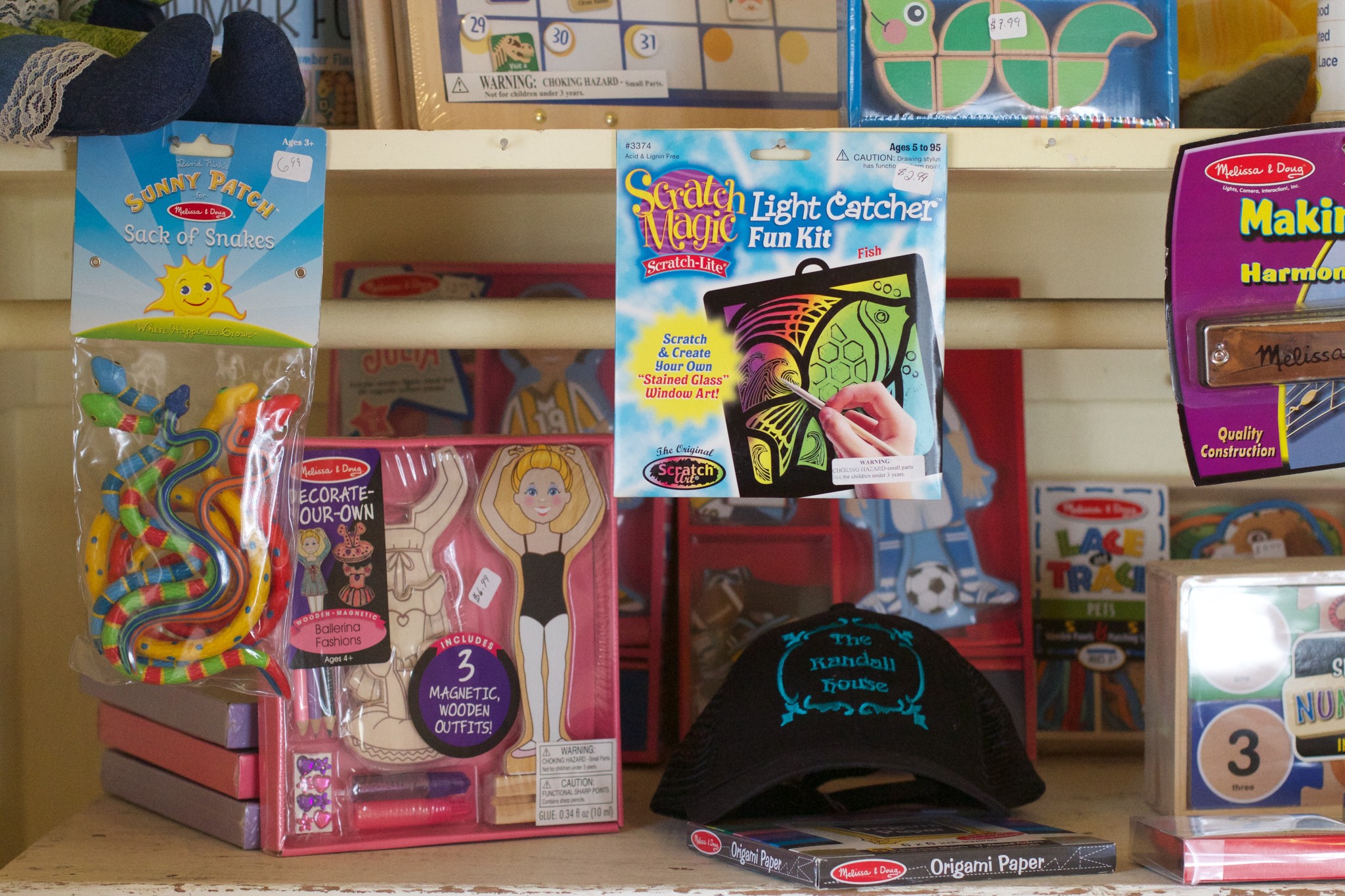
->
[818, 381, 916, 500]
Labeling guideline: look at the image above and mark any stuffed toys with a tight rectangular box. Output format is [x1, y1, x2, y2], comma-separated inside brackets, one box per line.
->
[0, 0, 307, 143]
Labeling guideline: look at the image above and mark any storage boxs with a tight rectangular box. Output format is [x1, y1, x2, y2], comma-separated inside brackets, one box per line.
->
[1142, 551, 1345, 829]
[259, 434, 628, 862]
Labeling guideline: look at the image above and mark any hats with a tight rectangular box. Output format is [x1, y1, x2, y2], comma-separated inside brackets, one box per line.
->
[648, 602, 1047, 827]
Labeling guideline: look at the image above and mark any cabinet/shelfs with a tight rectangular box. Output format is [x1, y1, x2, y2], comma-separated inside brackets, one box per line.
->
[0, 118, 1345, 896]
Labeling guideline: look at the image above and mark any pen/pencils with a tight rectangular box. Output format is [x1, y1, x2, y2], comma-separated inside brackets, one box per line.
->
[783, 378, 907, 464]
